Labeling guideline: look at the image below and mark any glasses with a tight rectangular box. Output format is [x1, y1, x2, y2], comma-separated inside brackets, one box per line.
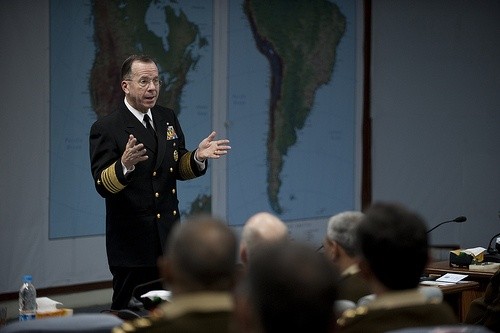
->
[125, 78, 164, 85]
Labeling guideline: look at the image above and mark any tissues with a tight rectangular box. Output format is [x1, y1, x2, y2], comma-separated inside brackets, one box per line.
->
[449, 247, 487, 269]
[35, 296, 73, 320]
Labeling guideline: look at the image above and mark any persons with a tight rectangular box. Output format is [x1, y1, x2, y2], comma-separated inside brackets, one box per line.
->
[90, 55, 232, 311]
[111, 200, 458, 333]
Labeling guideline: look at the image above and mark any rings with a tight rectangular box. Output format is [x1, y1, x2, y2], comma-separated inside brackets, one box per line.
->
[215, 150, 218, 154]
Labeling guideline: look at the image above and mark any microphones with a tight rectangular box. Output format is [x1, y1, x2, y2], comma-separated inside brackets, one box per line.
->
[427, 216, 467, 234]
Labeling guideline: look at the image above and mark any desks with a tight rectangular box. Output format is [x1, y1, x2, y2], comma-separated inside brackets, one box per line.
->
[425, 258, 500, 324]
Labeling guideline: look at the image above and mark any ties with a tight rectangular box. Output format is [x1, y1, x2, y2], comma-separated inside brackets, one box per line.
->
[143, 115, 158, 149]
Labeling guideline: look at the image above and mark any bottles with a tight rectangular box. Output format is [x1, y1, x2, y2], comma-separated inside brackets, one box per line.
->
[18, 275, 37, 322]
